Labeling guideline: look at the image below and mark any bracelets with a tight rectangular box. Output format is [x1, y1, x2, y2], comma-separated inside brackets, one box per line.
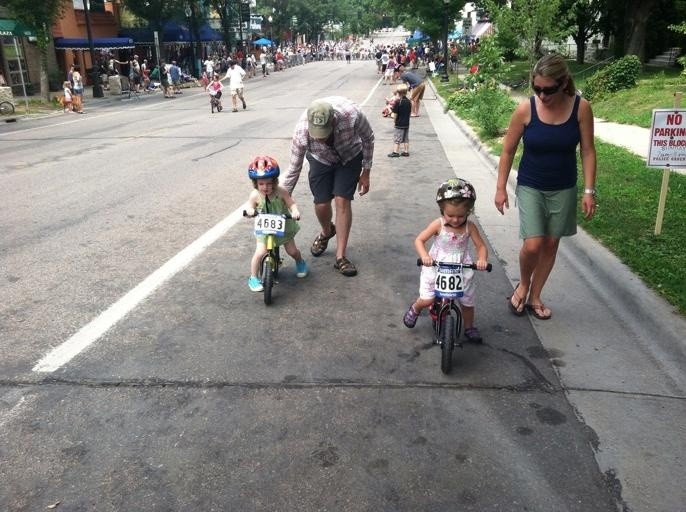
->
[584, 188, 597, 195]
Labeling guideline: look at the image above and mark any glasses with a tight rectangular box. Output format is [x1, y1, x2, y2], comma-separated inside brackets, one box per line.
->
[531, 79, 563, 95]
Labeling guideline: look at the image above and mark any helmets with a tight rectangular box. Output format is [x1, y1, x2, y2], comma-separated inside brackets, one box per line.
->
[248, 156, 280, 183]
[435, 177, 477, 205]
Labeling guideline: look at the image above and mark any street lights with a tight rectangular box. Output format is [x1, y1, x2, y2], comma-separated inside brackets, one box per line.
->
[267, 15, 274, 56]
[441, 0, 452, 82]
[183, 5, 197, 76]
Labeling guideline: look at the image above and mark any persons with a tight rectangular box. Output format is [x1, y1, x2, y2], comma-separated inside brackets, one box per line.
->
[279, 96, 375, 278]
[403, 177, 490, 343]
[386, 84, 412, 158]
[494, 52, 598, 322]
[241, 156, 310, 293]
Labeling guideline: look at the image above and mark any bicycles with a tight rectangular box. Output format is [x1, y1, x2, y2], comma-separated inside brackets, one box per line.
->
[0, 101, 15, 116]
[383, 97, 396, 119]
[242, 212, 298, 304]
[414, 257, 493, 370]
[207, 88, 223, 114]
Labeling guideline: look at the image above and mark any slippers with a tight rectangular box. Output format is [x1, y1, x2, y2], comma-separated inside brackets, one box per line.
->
[510, 281, 528, 316]
[524, 298, 552, 321]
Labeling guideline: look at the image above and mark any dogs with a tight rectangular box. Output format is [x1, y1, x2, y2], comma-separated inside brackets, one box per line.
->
[54, 95, 83, 113]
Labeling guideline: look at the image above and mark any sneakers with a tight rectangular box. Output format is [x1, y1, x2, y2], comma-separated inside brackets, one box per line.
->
[294, 260, 307, 278]
[231, 104, 248, 113]
[386, 151, 401, 158]
[401, 151, 410, 157]
[247, 274, 265, 293]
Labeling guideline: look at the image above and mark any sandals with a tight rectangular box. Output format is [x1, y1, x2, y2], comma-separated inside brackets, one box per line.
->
[402, 300, 422, 328]
[310, 224, 336, 257]
[331, 255, 358, 277]
[462, 326, 483, 344]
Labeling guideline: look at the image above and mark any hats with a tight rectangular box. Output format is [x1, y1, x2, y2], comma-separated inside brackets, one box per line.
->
[306, 103, 335, 140]
